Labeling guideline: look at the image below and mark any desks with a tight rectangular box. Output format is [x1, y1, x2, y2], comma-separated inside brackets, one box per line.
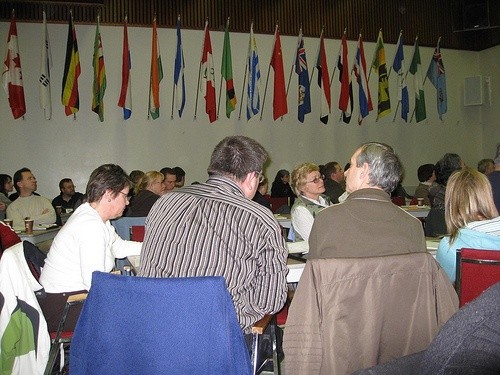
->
[127, 237, 441, 282]
[278, 206, 431, 228]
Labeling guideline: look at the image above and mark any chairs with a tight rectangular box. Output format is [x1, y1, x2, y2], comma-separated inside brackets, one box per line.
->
[455, 248, 500, 308]
[3, 239, 281, 375]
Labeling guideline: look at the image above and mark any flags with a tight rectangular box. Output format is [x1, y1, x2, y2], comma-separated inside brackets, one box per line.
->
[173, 22, 186, 117]
[221, 26, 238, 119]
[0, 16, 26, 120]
[200, 26, 216, 124]
[61, 12, 81, 116]
[38, 13, 52, 120]
[91, 21, 107, 122]
[149, 20, 164, 119]
[295, 32, 447, 125]
[245, 29, 261, 121]
[270, 29, 288, 121]
[118, 21, 133, 120]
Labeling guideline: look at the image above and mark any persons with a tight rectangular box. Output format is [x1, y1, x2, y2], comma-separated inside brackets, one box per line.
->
[308, 142, 427, 258]
[436, 169, 500, 286]
[0, 168, 87, 255]
[136, 135, 287, 375]
[350, 282, 500, 375]
[253, 162, 350, 259]
[122, 166, 186, 217]
[35, 164, 145, 375]
[392, 147, 500, 237]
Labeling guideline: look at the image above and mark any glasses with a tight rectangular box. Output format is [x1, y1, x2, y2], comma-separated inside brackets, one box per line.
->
[64, 185, 76, 189]
[255, 171, 265, 183]
[307, 174, 325, 184]
[119, 191, 132, 201]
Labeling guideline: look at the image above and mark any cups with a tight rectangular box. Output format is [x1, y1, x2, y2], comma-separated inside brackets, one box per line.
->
[56, 206, 62, 215]
[417, 197, 424, 206]
[406, 199, 411, 206]
[24, 220, 33, 233]
[4, 218, 12, 228]
